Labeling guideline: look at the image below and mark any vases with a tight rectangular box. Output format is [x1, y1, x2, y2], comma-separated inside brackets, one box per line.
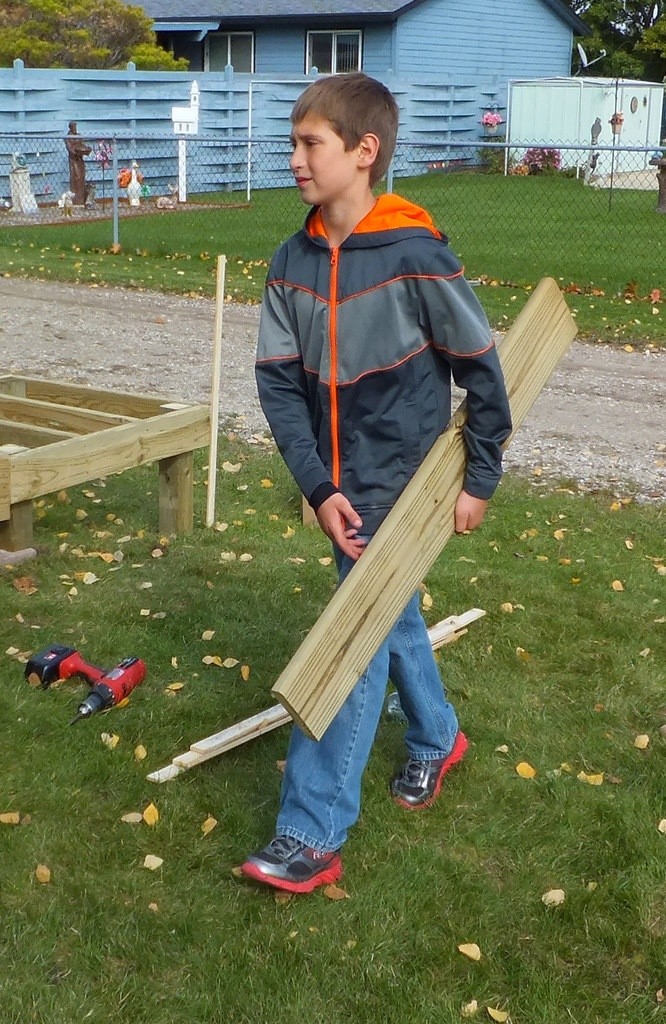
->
[485, 125, 499, 134]
[127, 169, 143, 206]
[612, 124, 622, 134]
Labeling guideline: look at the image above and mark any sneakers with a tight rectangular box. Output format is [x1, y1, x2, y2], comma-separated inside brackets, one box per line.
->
[390, 727, 468, 811]
[240, 834, 343, 893]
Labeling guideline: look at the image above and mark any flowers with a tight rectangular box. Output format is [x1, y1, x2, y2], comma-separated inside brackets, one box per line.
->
[610, 112, 625, 134]
[481, 112, 503, 135]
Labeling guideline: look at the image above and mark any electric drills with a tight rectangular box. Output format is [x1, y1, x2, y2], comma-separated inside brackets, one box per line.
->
[23, 642, 150, 730]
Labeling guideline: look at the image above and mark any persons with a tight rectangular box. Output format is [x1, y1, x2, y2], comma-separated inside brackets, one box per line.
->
[65, 121, 94, 205]
[241, 74, 513, 893]
